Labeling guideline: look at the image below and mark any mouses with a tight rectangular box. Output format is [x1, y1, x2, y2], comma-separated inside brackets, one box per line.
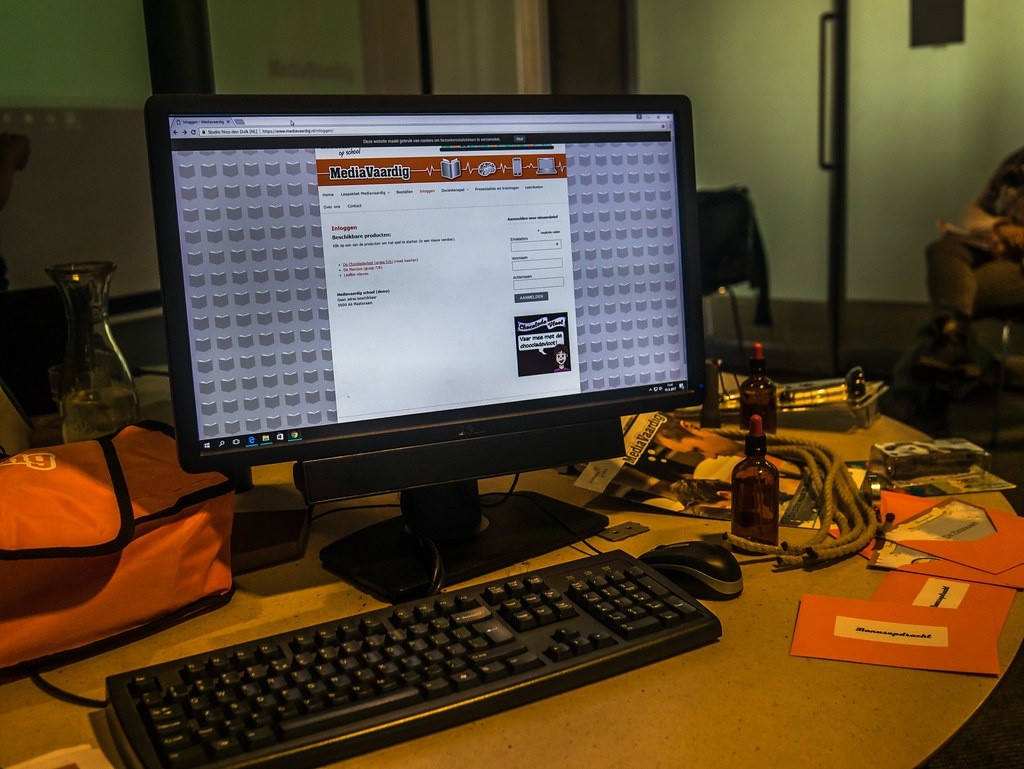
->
[638, 540, 744, 601]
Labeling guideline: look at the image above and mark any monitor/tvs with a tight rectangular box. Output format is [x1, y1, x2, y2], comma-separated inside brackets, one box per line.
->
[144, 91, 706, 604]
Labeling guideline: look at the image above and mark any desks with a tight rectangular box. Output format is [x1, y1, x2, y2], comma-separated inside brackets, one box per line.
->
[0, 363, 1024, 769]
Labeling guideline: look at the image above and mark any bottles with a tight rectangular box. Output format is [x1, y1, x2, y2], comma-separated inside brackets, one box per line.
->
[740, 342, 776, 435]
[46, 260, 138, 443]
[731, 415, 778, 548]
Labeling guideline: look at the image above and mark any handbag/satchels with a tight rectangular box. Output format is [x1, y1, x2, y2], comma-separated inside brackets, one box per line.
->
[0, 420, 235, 686]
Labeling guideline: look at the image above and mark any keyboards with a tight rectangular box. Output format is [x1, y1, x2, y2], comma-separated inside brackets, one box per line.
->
[106, 550, 722, 769]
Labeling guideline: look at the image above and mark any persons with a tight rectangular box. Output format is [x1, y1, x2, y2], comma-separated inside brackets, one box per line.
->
[652, 414, 802, 522]
[890, 145, 1024, 440]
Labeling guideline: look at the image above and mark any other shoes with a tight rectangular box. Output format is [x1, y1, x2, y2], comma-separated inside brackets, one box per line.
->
[919, 309, 973, 374]
